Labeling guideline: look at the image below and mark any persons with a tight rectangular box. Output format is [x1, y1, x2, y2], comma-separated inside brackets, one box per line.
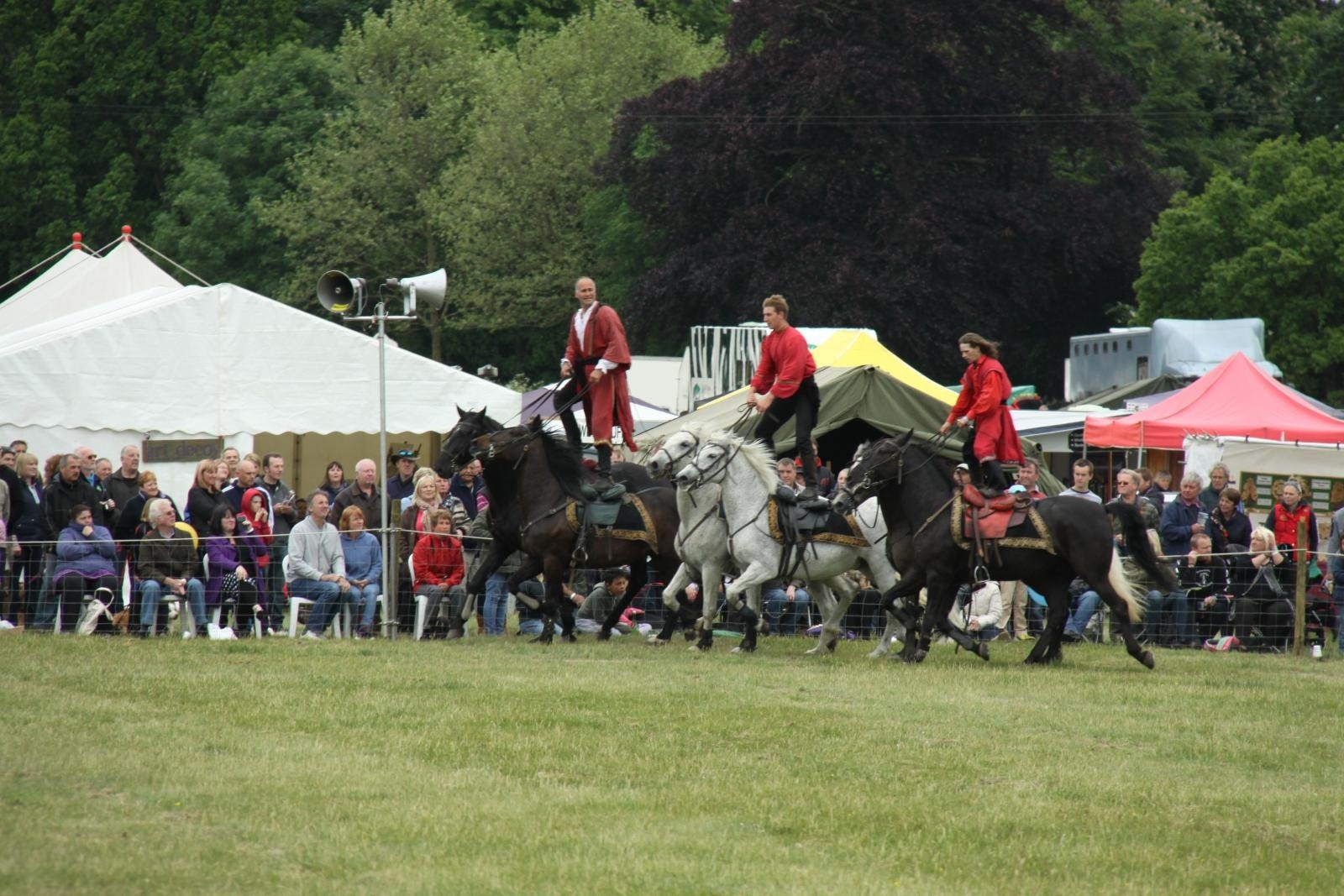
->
[0, 440, 1344, 658]
[940, 332, 1024, 495]
[746, 294, 817, 501]
[553, 275, 637, 479]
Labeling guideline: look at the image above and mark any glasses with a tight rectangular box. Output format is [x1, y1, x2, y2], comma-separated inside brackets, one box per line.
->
[88, 455, 97, 460]
[160, 510, 177, 516]
[1118, 481, 1137, 487]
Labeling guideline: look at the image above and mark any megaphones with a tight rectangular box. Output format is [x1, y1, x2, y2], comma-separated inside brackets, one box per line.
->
[316, 270, 366, 314]
[386, 267, 447, 310]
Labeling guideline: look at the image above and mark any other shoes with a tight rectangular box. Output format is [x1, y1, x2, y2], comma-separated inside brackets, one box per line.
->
[798, 487, 819, 500]
[591, 475, 615, 489]
[2, 617, 464, 642]
[982, 485, 1003, 499]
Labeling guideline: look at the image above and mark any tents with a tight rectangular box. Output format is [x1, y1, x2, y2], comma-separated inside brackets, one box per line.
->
[0, 225, 1344, 558]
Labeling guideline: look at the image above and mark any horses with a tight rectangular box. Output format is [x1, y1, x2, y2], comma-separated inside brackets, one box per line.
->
[677, 429, 898, 658]
[833, 428, 1157, 668]
[469, 412, 679, 641]
[436, 403, 678, 647]
[646, 420, 900, 657]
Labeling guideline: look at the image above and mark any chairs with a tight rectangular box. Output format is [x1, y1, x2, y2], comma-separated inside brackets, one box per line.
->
[55, 552, 470, 640]
[1222, 539, 1294, 653]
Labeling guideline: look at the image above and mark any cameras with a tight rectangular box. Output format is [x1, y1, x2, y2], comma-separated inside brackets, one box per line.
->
[237, 515, 247, 533]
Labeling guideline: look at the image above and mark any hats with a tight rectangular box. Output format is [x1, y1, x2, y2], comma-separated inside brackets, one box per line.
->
[0, 447, 18, 456]
[955, 463, 970, 471]
[392, 449, 423, 466]
